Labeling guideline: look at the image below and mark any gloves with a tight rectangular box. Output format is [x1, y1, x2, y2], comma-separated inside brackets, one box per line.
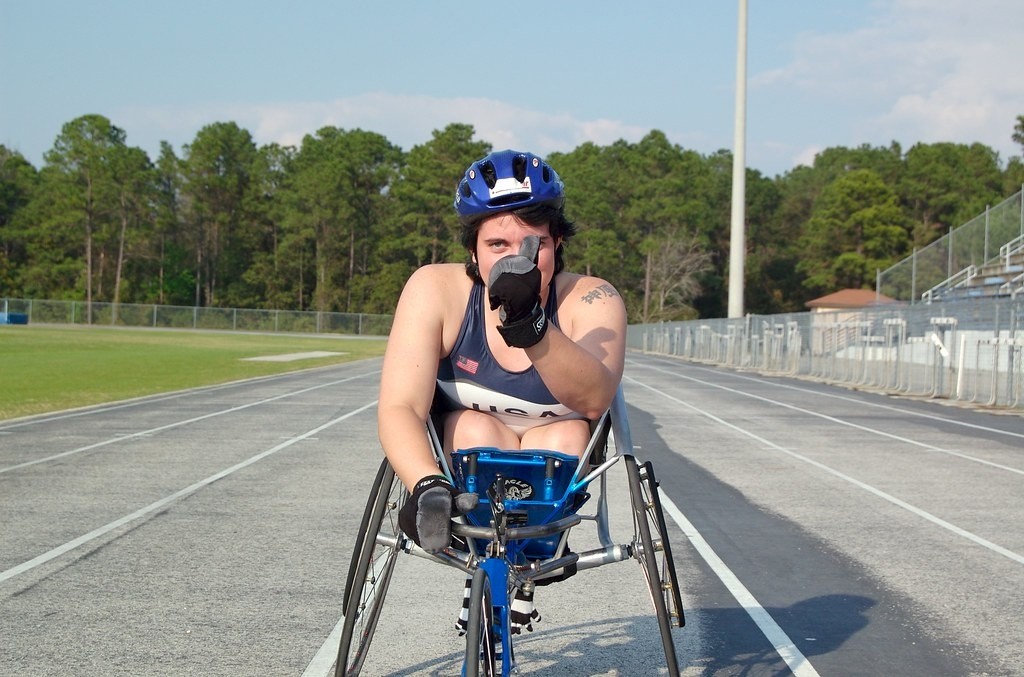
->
[487, 236, 548, 348]
[510, 589, 541, 635]
[398, 475, 478, 553]
[454, 579, 471, 639]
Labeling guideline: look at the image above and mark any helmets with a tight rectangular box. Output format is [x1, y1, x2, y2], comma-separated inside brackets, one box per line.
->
[453, 149, 565, 226]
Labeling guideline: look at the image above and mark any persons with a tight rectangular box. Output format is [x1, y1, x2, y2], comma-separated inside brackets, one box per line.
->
[377, 149, 628, 555]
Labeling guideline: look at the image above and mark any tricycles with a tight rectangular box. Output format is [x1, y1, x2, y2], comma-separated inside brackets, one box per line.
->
[334, 384, 685, 677]
